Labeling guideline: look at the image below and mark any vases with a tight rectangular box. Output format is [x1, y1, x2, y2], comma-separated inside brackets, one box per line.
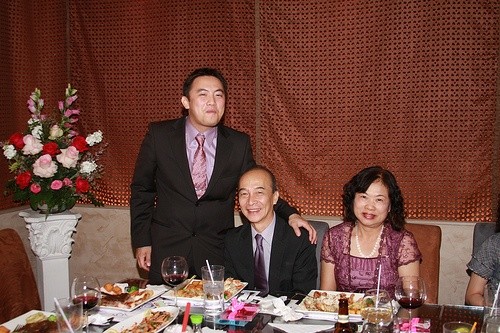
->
[30, 194, 78, 214]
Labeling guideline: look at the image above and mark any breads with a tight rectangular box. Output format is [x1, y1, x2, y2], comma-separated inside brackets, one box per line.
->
[304, 297, 338, 313]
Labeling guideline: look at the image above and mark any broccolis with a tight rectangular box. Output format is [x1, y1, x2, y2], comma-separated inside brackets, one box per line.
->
[127, 283, 139, 293]
[48, 315, 57, 322]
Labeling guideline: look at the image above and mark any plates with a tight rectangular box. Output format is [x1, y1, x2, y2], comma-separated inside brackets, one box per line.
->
[295, 290, 366, 318]
[103, 305, 181, 333]
[1, 309, 95, 332]
[160, 278, 249, 303]
[66, 285, 171, 311]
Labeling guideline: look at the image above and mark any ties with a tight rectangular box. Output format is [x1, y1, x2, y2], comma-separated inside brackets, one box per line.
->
[191, 134, 208, 199]
[253, 234, 269, 297]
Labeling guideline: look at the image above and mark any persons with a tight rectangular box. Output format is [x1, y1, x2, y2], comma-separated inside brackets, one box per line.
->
[466, 201, 500, 306]
[320, 166, 422, 298]
[130, 67, 318, 286]
[222, 165, 318, 295]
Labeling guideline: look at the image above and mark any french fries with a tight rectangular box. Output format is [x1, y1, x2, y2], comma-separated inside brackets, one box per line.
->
[348, 294, 367, 311]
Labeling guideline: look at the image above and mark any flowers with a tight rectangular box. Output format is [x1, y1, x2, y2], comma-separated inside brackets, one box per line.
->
[0, 84, 108, 220]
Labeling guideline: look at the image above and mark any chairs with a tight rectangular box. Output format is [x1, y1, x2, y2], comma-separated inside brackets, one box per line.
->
[405, 223, 442, 305]
[0, 228, 43, 327]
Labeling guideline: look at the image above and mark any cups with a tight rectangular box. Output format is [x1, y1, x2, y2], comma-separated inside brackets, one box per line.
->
[442, 321, 476, 333]
[360, 288, 393, 333]
[484, 283, 500, 333]
[481, 315, 500, 333]
[201, 265, 225, 315]
[163, 325, 192, 333]
[54, 298, 84, 333]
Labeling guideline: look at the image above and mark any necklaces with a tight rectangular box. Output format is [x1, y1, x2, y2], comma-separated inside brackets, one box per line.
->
[355, 220, 384, 258]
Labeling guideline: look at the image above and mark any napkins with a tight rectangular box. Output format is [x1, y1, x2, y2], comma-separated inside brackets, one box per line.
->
[268, 323, 334, 333]
[272, 298, 304, 321]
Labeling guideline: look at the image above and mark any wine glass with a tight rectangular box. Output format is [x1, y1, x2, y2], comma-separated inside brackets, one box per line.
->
[394, 276, 427, 322]
[72, 275, 101, 333]
[161, 256, 189, 309]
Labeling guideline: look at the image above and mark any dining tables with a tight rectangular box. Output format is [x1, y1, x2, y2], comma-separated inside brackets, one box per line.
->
[50, 279, 487, 333]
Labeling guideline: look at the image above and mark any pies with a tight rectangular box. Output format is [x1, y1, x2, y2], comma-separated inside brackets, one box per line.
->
[11, 320, 59, 333]
[102, 290, 153, 308]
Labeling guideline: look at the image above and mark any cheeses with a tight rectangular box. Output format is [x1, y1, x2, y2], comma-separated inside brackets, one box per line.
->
[224, 278, 245, 295]
[27, 312, 48, 324]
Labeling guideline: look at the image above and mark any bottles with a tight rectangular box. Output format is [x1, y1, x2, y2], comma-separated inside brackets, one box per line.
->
[190, 314, 203, 333]
[334, 298, 353, 333]
